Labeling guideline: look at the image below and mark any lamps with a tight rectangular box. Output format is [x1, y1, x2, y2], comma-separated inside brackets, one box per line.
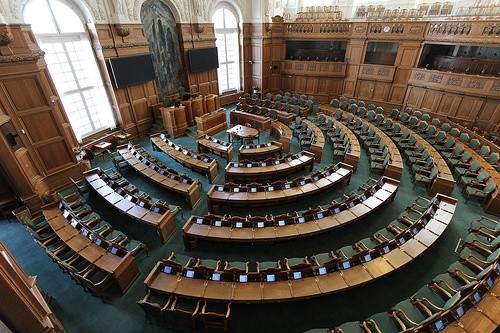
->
[7, 128, 24, 147]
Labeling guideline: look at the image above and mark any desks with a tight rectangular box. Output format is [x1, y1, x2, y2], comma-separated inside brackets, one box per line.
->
[403, 112, 500, 216]
[117, 133, 131, 140]
[318, 113, 360, 171]
[438, 261, 500, 333]
[144, 194, 458, 304]
[271, 119, 291, 153]
[239, 96, 310, 118]
[208, 163, 353, 214]
[237, 102, 293, 126]
[319, 105, 403, 182]
[94, 142, 111, 151]
[237, 139, 282, 162]
[84, 167, 177, 245]
[196, 134, 234, 161]
[300, 117, 325, 160]
[117, 144, 201, 211]
[152, 135, 219, 184]
[235, 126, 258, 143]
[42, 203, 142, 295]
[447, 119, 500, 163]
[331, 100, 456, 196]
[224, 150, 315, 183]
[195, 108, 227, 132]
[230, 108, 272, 132]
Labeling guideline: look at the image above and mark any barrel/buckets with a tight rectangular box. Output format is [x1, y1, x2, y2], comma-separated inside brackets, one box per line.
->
[46, 190, 58, 202]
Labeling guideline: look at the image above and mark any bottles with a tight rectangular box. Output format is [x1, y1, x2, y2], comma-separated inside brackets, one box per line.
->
[240, 127, 242, 131]
[237, 128, 238, 132]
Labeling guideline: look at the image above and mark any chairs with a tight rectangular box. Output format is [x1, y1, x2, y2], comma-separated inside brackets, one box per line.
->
[19, 86, 500, 333]
